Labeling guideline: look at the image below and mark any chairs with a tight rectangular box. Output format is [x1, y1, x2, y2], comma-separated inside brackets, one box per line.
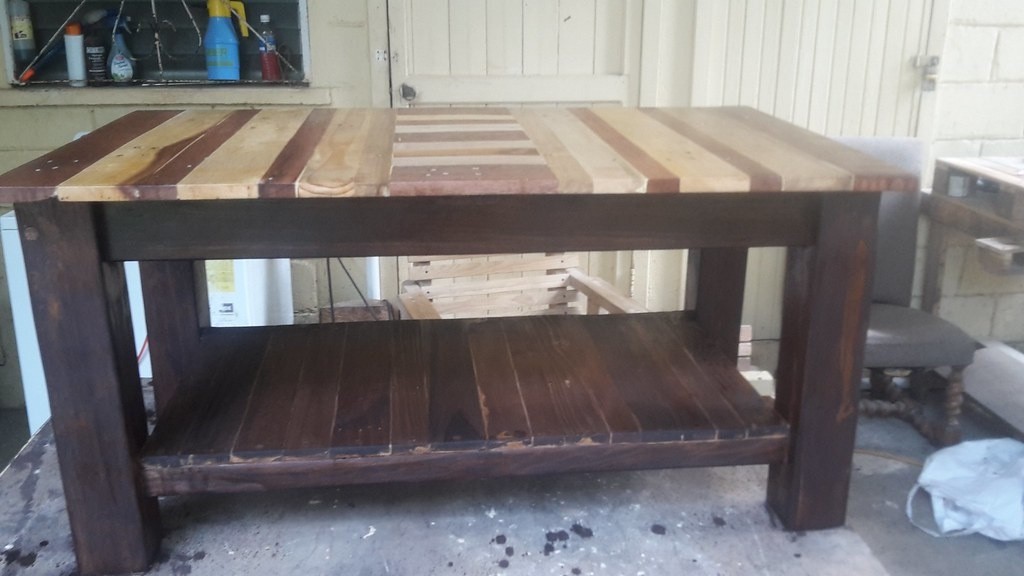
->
[401, 248, 649, 318]
[829, 134, 987, 452]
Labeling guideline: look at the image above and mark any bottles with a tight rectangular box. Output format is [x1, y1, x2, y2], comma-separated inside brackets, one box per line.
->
[259, 15, 281, 81]
[63, 23, 87, 88]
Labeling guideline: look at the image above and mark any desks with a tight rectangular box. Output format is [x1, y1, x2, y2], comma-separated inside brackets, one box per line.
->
[1, 108, 924, 575]
[918, 155, 1024, 440]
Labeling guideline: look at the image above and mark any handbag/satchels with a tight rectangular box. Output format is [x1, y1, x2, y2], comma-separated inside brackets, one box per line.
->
[902, 437, 1023, 543]
